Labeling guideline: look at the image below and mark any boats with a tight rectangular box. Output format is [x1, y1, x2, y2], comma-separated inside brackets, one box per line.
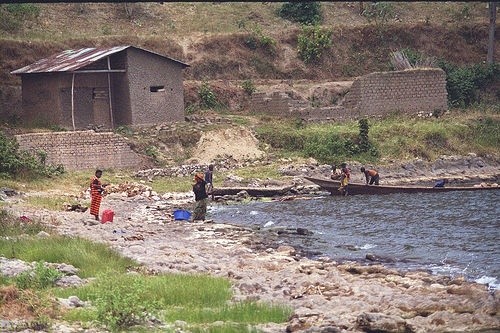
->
[303, 176, 500, 197]
[209, 182, 296, 196]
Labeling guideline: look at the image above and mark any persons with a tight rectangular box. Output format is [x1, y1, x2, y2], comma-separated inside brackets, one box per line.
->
[90, 169, 107, 221]
[435, 178, 448, 187]
[361, 167, 379, 185]
[205, 164, 215, 201]
[192, 172, 209, 222]
[331, 162, 351, 197]
